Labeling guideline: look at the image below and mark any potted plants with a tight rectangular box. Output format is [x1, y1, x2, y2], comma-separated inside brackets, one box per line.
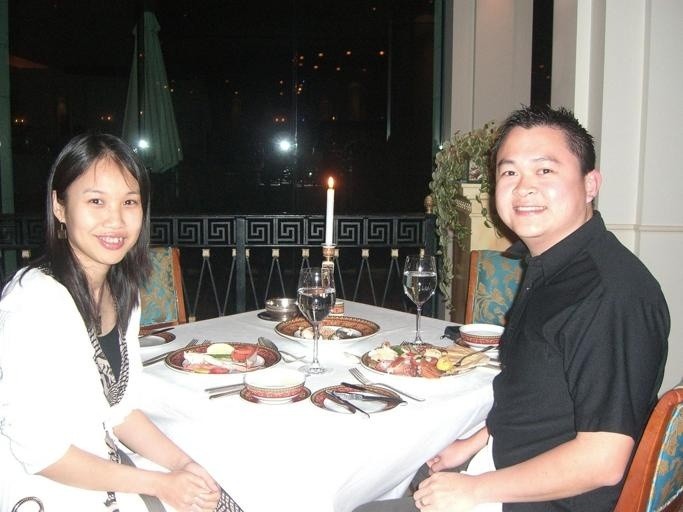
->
[429, 121, 513, 313]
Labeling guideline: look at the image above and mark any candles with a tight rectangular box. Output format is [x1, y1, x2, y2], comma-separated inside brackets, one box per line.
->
[325, 176, 335, 247]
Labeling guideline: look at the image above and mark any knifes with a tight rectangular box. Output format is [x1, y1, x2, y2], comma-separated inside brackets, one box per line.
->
[138, 327, 174, 338]
[325, 382, 408, 418]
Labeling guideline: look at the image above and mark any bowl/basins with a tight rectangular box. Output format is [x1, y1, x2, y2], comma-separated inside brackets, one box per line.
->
[459, 324, 504, 350]
[245, 370, 305, 402]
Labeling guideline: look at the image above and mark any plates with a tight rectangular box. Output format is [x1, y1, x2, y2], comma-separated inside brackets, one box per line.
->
[166, 343, 282, 375]
[240, 387, 401, 416]
[257, 297, 381, 347]
[139, 330, 176, 348]
[361, 345, 486, 379]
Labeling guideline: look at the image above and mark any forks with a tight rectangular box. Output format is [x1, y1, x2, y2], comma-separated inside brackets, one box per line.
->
[347, 368, 426, 401]
[140, 339, 212, 366]
[454, 346, 495, 367]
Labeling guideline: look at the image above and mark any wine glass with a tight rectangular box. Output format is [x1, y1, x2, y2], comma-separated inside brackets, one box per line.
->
[296, 268, 337, 377]
[403, 255, 437, 344]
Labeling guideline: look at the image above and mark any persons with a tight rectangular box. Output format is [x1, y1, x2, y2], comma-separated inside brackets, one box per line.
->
[351, 104, 671, 512]
[0, 133, 222, 511]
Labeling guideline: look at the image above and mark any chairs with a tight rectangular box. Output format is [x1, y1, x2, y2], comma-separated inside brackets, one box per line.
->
[613, 388, 683, 512]
[137, 246, 186, 335]
[463, 249, 527, 328]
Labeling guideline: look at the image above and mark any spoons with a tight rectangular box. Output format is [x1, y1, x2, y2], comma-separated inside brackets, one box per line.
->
[257, 337, 306, 363]
